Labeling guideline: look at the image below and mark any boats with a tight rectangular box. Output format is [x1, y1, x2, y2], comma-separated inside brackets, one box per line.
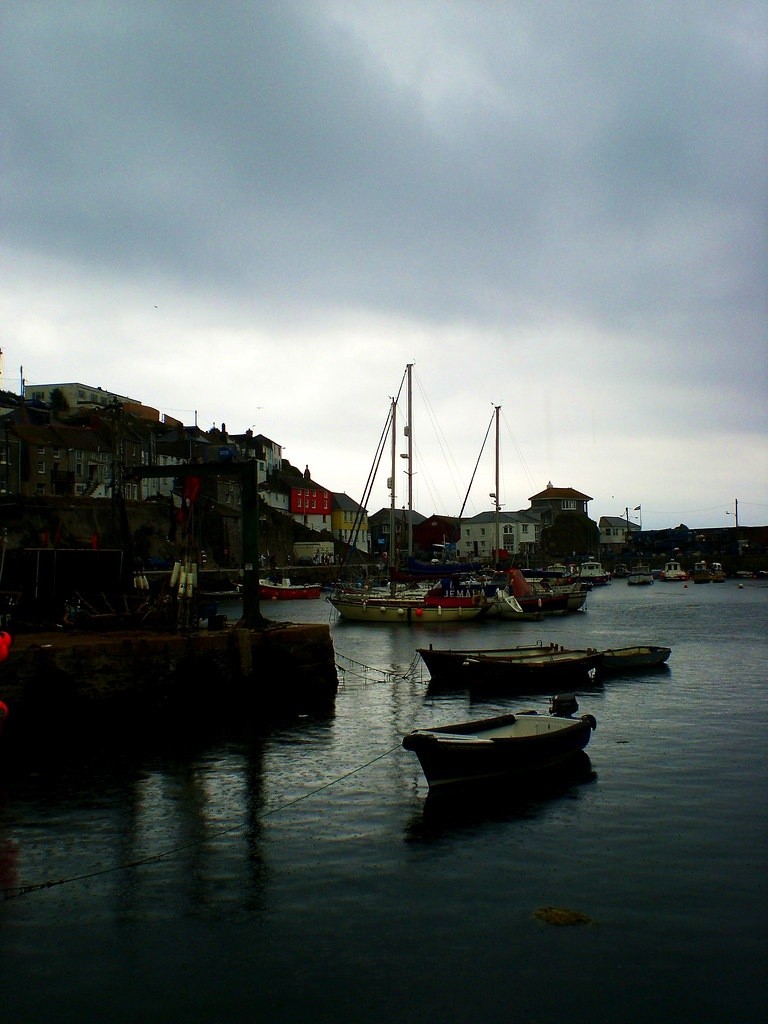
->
[401, 693, 599, 793]
[461, 645, 672, 685]
[259, 578, 323, 600]
[414, 640, 571, 688]
[323, 355, 725, 622]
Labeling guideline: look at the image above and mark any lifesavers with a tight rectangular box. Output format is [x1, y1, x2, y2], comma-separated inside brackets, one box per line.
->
[472, 595, 483, 608]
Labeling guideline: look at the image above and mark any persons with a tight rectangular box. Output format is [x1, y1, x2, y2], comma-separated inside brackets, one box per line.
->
[313, 549, 344, 566]
[259, 554, 266, 568]
[270, 555, 277, 572]
[287, 553, 291, 568]
